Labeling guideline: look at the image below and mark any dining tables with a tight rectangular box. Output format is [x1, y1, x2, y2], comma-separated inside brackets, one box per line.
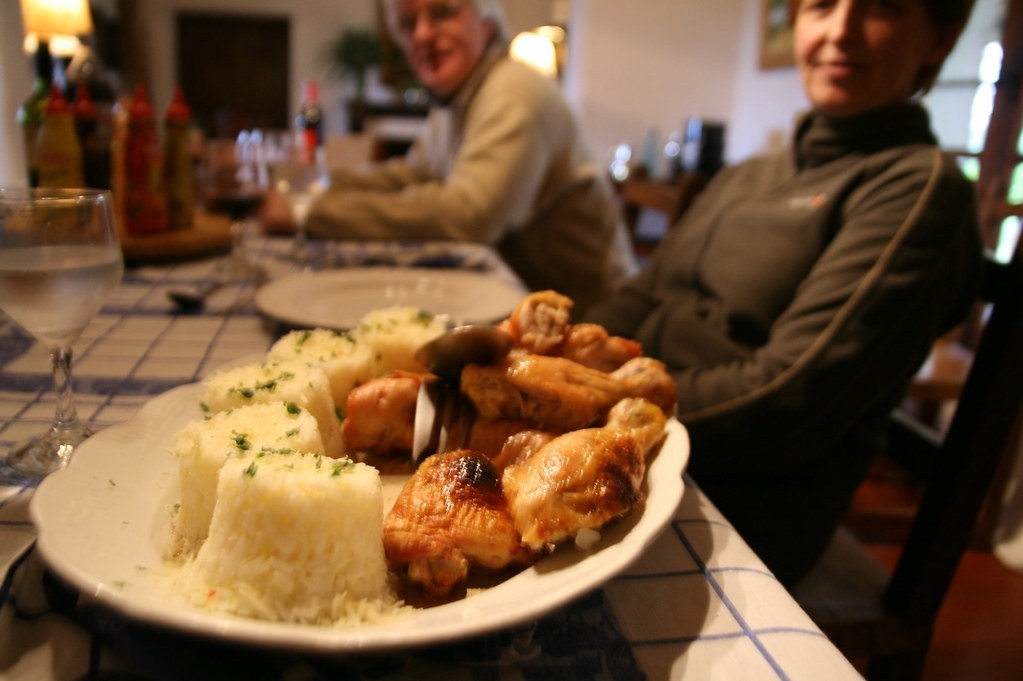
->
[0, 168, 866, 681]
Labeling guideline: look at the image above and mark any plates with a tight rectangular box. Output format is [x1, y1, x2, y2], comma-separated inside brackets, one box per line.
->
[25, 375, 692, 654]
[254, 264, 525, 330]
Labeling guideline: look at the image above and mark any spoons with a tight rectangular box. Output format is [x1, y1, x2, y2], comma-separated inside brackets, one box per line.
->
[163, 264, 226, 313]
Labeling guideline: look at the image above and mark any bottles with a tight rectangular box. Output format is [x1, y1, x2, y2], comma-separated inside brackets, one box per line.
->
[24, 71, 198, 236]
[296, 79, 322, 164]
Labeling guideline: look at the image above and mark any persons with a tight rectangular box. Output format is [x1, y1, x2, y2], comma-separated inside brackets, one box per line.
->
[262, 0, 639, 325]
[572, 1, 973, 586]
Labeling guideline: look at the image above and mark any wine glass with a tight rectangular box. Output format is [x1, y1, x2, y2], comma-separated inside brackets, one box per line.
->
[273, 160, 333, 262]
[1, 186, 125, 477]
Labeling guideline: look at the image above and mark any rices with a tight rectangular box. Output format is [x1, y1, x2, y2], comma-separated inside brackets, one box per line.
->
[156, 308, 457, 628]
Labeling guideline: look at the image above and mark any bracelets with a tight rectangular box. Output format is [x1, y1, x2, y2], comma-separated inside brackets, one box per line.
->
[290, 194, 311, 227]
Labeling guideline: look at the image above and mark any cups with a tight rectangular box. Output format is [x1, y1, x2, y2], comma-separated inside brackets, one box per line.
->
[232, 125, 289, 186]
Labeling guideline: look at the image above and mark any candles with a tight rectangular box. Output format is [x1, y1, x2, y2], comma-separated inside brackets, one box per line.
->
[19, 0, 94, 40]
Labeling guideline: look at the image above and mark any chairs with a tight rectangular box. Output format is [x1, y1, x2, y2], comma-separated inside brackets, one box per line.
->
[779, 230, 1023, 681]
[619, 163, 697, 267]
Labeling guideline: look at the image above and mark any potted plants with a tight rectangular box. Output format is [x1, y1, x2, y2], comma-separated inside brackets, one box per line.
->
[318, 27, 391, 133]
